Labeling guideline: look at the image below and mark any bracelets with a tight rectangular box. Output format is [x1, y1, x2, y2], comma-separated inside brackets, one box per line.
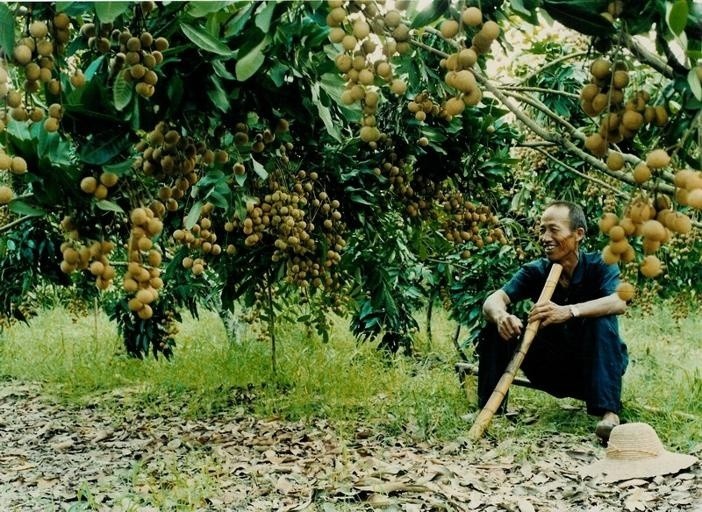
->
[569, 306, 578, 317]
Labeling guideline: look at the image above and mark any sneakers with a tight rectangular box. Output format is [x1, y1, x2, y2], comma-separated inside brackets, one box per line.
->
[595, 415, 620, 441]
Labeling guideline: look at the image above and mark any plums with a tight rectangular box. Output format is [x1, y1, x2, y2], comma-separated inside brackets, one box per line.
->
[0, 0, 702, 319]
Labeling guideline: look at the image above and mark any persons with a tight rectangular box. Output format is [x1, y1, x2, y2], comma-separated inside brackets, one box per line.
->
[476, 204, 631, 439]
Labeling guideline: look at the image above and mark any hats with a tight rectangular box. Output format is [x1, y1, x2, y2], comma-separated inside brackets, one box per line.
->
[577, 422, 698, 484]
